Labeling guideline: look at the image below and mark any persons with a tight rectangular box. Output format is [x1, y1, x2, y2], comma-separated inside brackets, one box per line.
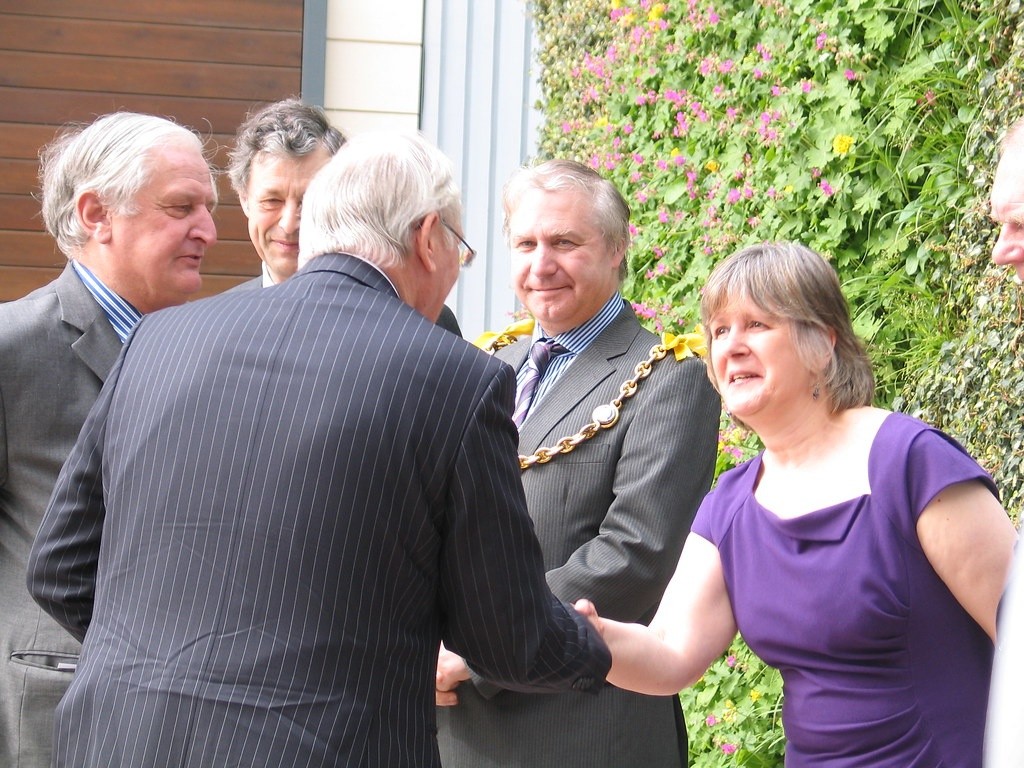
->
[223, 98, 461, 341]
[2, 112, 217, 767]
[572, 240, 1017, 768]
[983, 115, 1023, 768]
[436, 156, 722, 768]
[28, 131, 612, 768]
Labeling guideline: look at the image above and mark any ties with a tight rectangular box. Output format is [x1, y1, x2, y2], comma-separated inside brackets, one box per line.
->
[511, 340, 569, 428]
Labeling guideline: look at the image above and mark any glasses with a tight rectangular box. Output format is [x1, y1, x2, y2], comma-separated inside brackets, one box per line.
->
[440, 218, 476, 267]
